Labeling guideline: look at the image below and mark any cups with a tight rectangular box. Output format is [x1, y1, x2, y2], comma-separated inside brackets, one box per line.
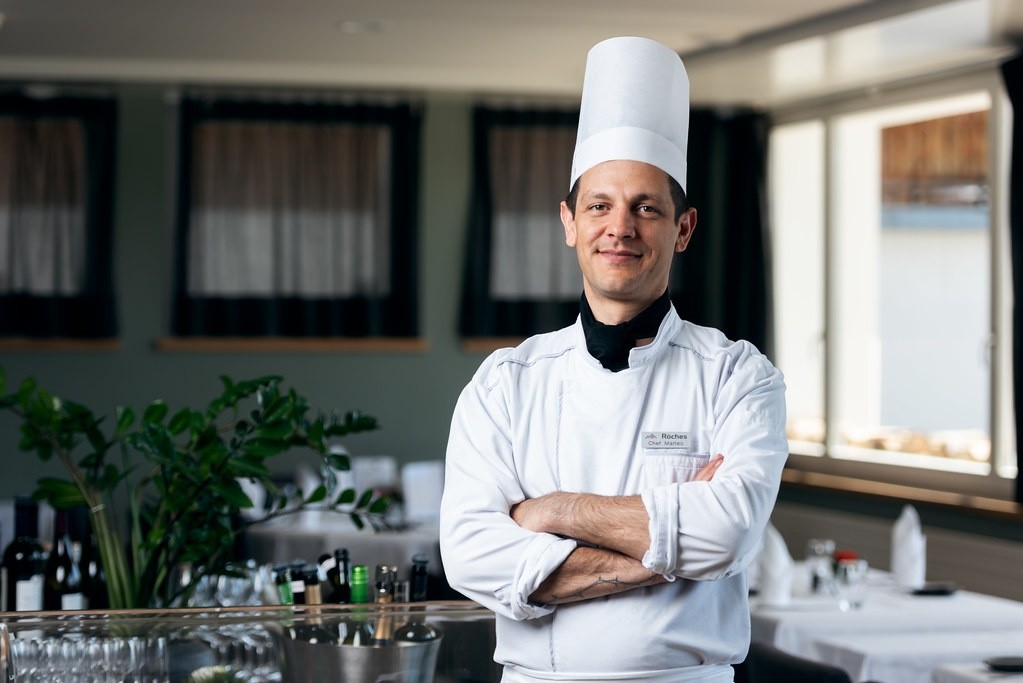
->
[809, 539, 835, 576]
[8, 636, 170, 682]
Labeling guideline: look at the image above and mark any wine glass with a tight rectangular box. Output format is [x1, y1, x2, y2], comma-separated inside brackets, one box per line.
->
[838, 559, 872, 636]
[184, 562, 285, 683]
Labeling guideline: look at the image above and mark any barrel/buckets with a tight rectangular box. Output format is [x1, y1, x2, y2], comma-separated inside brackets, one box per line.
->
[276, 631, 443, 683]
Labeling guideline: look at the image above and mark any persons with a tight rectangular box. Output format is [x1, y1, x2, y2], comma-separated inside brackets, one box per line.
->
[439, 37, 788, 683]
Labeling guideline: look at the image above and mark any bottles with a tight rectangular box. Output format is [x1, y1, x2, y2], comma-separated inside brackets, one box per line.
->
[51, 501, 105, 637]
[276, 547, 436, 649]
[3, 499, 53, 640]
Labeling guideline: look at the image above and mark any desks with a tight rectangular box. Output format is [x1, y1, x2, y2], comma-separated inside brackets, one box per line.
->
[746, 566, 1023, 683]
[231, 507, 442, 579]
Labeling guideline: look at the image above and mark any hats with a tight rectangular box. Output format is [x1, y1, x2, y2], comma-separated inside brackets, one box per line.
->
[569, 36, 690, 199]
